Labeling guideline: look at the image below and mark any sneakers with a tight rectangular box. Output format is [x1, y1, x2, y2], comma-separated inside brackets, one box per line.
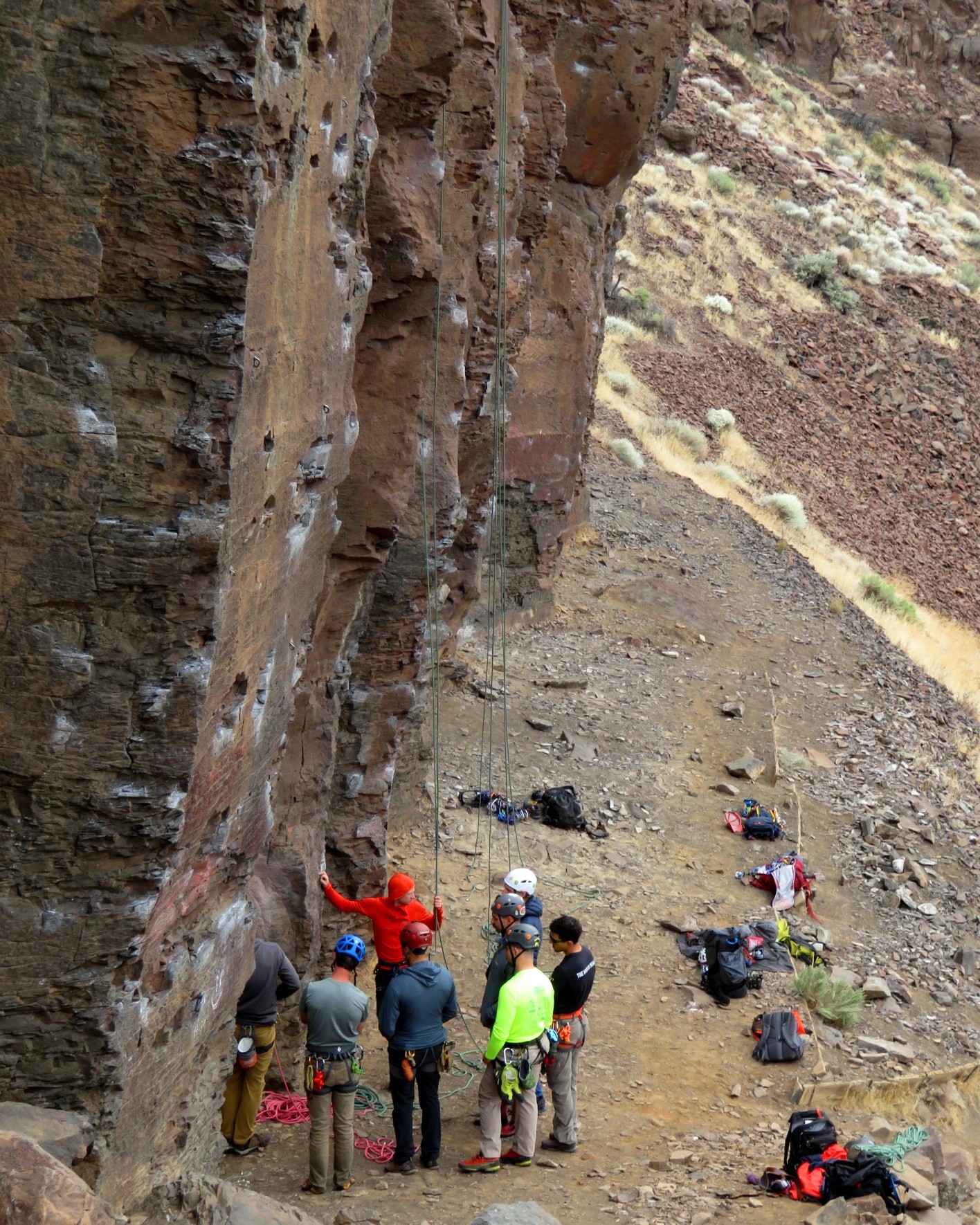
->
[499, 1148, 532, 1166]
[458, 1149, 500, 1172]
[541, 1134, 577, 1152]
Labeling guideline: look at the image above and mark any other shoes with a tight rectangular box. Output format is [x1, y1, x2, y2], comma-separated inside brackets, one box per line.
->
[334, 1174, 356, 1191]
[234, 1130, 271, 1156]
[760, 1168, 790, 1197]
[745, 944, 764, 967]
[508, 1097, 546, 1112]
[414, 1153, 441, 1169]
[746, 971, 764, 989]
[390, 1157, 418, 1174]
[302, 1180, 326, 1194]
[500, 1120, 519, 1137]
[584, 824, 605, 837]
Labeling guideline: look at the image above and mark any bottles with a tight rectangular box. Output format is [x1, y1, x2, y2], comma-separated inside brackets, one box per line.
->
[742, 798, 752, 817]
[848, 1144, 861, 1159]
[772, 1179, 789, 1194]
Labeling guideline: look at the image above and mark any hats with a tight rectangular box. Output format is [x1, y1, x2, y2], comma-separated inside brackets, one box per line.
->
[724, 810, 742, 833]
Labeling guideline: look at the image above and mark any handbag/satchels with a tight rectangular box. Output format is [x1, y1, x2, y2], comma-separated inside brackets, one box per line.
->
[703, 925, 747, 1000]
[541, 784, 581, 831]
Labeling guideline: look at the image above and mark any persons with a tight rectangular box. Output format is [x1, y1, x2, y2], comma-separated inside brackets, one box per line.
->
[376, 922, 461, 1176]
[221, 937, 300, 1155]
[317, 870, 444, 1021]
[298, 934, 370, 1195]
[457, 867, 596, 1173]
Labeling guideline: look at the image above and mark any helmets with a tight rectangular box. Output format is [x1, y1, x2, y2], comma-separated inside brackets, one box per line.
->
[490, 893, 526, 918]
[505, 923, 541, 948]
[336, 933, 366, 962]
[504, 868, 537, 895]
[399, 922, 434, 948]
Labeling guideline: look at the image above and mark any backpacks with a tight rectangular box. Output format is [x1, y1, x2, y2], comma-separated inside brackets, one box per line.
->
[819, 1149, 901, 1217]
[754, 1010, 804, 1065]
[781, 1108, 837, 1172]
[744, 814, 778, 841]
[755, 857, 806, 895]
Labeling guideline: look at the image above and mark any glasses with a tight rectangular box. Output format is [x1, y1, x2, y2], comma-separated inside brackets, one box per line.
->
[550, 934, 563, 944]
